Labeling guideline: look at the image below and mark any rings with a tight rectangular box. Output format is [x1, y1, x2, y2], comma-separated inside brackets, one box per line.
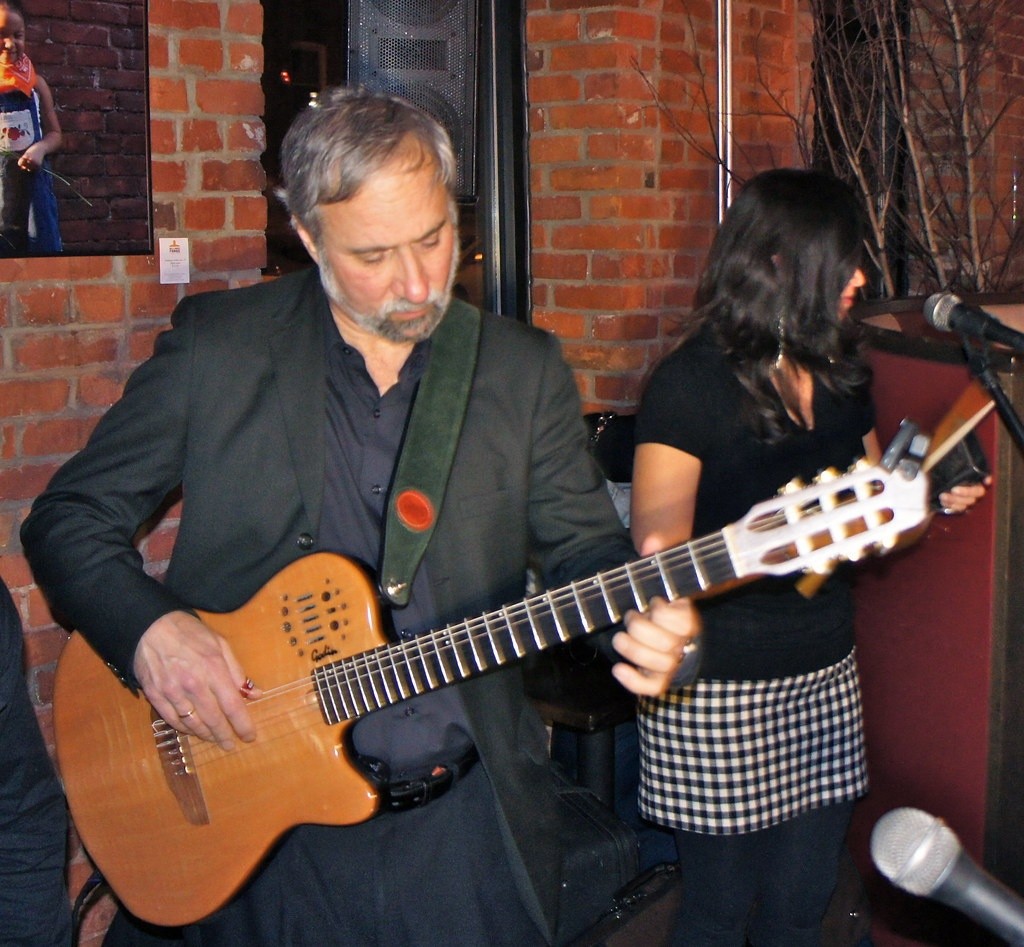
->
[239, 676, 253, 697]
[180, 708, 195, 721]
[679, 637, 689, 665]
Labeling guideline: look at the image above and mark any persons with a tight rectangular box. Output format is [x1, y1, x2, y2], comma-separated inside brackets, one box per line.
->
[21, 90, 698, 947]
[0, 563, 68, 947]
[632, 170, 991, 947]
[0, 3, 62, 252]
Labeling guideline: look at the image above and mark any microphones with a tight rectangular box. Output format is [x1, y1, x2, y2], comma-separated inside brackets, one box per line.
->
[871, 806, 1023, 946]
[923, 293, 1024, 353]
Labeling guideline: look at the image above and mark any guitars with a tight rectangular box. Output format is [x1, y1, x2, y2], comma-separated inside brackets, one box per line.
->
[48, 421, 937, 931]
